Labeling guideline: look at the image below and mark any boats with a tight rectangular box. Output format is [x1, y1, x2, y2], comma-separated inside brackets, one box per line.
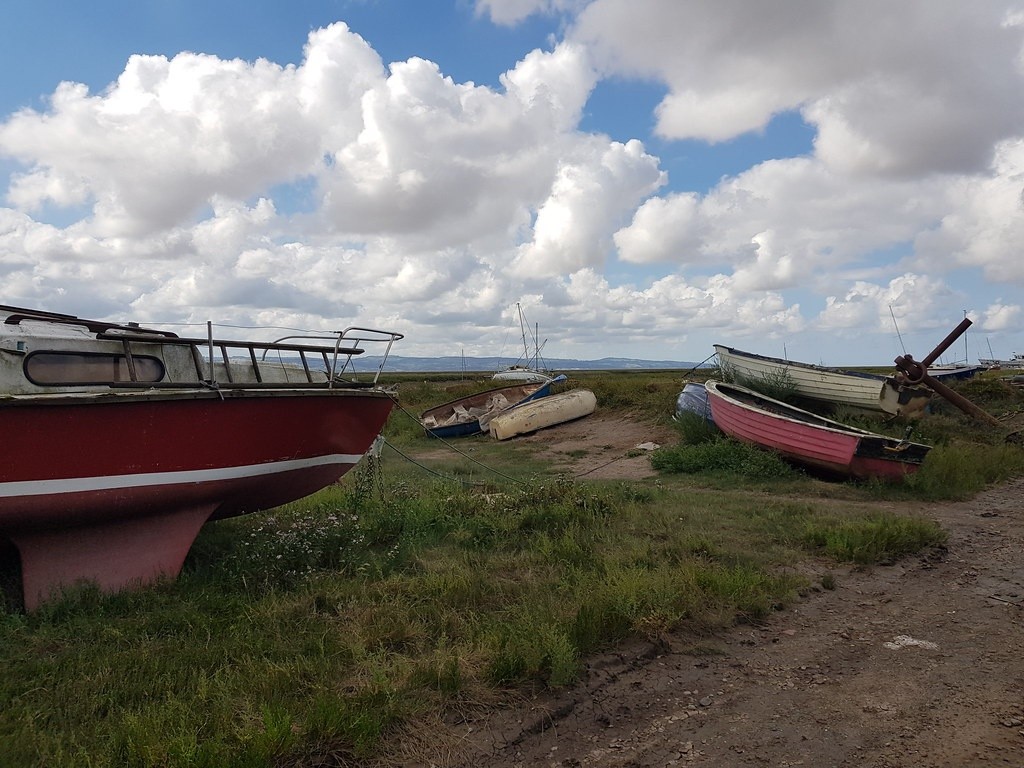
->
[978, 355, 1024, 367]
[421, 374, 568, 439]
[677, 383, 716, 422]
[712, 344, 934, 427]
[705, 379, 934, 485]
[0, 305, 400, 616]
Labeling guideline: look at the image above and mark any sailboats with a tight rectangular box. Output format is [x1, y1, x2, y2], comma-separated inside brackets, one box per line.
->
[887, 305, 978, 382]
[491, 303, 554, 387]
